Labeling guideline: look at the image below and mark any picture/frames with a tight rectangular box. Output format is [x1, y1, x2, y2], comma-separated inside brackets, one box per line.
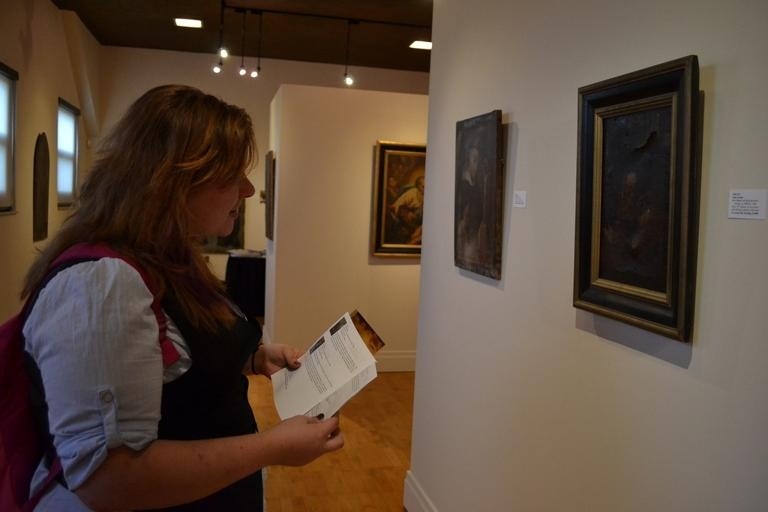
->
[450, 109, 503, 282]
[264, 152, 275, 241]
[574, 55, 701, 341]
[373, 140, 423, 261]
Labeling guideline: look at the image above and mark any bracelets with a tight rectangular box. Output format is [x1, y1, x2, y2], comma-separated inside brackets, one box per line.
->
[251, 343, 264, 375]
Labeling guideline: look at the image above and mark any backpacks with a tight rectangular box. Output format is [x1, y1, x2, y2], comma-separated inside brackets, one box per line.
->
[0, 245, 165, 512]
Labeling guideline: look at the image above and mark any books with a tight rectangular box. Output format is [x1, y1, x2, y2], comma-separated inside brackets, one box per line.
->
[270, 311, 378, 421]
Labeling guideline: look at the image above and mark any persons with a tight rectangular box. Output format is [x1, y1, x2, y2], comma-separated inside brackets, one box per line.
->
[386, 163, 424, 243]
[18, 84, 345, 512]
[459, 147, 492, 243]
